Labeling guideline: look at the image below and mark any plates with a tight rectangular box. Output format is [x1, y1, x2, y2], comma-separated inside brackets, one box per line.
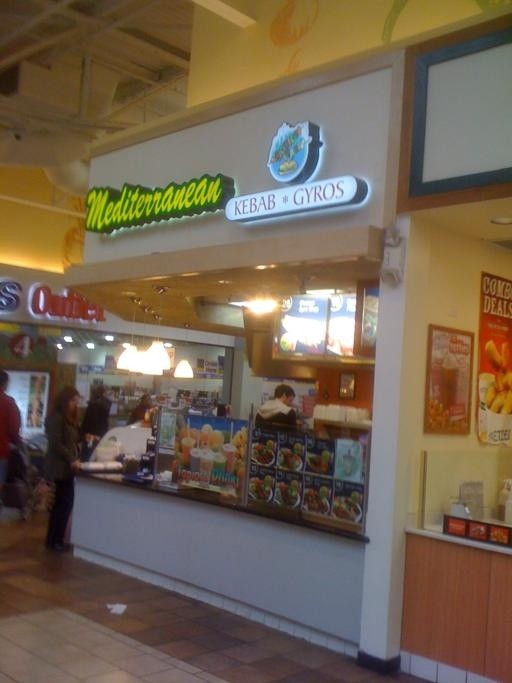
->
[247, 443, 362, 524]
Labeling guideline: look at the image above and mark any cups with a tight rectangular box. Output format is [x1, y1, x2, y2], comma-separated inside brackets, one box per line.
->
[440, 363, 459, 410]
[486, 407, 512, 445]
[180, 435, 238, 489]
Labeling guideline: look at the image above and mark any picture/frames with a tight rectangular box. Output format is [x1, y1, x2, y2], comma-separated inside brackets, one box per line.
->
[422, 323, 475, 437]
[336, 370, 359, 401]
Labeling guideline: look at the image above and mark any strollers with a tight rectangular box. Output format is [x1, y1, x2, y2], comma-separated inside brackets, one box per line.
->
[3, 435, 57, 521]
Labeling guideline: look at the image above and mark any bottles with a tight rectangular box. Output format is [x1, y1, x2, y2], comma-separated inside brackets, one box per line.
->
[477, 372, 495, 443]
[123, 450, 138, 474]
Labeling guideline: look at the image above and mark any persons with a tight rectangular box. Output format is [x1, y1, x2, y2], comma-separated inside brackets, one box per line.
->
[79, 384, 112, 472]
[255, 385, 297, 426]
[127, 393, 152, 424]
[45, 388, 92, 552]
[0, 370, 23, 503]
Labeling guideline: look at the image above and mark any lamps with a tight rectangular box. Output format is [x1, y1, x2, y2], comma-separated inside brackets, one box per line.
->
[115, 294, 194, 379]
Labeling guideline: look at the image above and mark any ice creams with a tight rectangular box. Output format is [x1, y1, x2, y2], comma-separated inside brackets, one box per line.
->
[440, 355, 459, 407]
